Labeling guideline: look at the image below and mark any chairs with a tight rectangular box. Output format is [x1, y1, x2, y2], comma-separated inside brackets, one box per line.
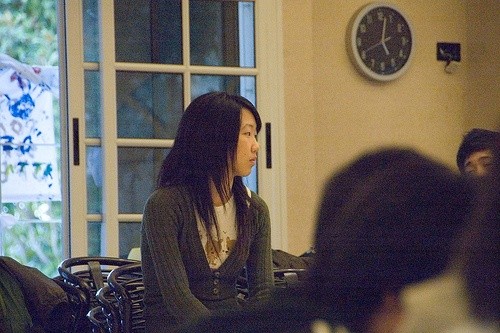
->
[54, 256, 148, 333]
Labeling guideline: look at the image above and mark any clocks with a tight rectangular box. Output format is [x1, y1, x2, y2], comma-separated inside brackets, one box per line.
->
[346, 2, 415, 82]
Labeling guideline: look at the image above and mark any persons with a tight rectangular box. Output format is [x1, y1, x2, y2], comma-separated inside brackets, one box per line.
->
[316, 127, 500, 333]
[140, 92, 275, 333]
